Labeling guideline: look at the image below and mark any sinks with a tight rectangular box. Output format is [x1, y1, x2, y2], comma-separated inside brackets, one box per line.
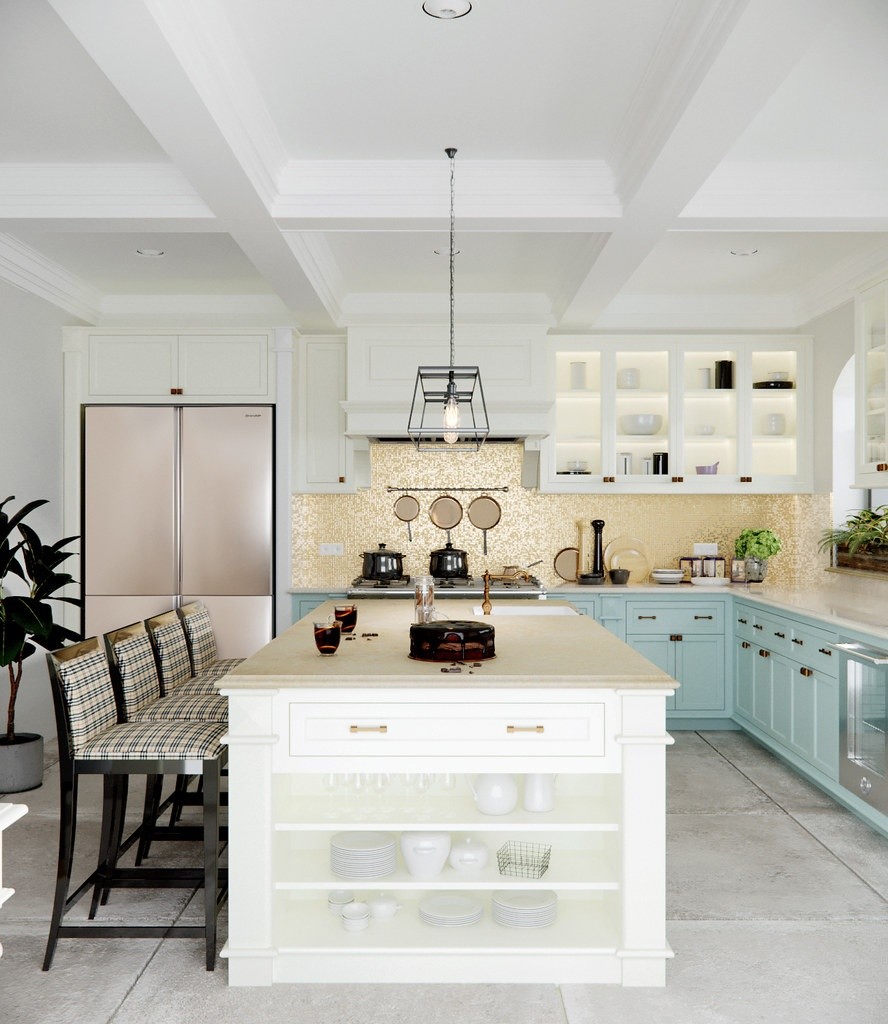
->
[473, 605, 581, 616]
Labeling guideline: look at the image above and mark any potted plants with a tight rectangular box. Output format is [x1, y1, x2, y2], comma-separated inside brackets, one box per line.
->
[817, 504, 888, 572]
[735, 528, 782, 582]
[0, 495, 84, 794]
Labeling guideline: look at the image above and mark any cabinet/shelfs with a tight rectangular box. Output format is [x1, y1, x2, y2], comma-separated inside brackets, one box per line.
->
[536, 333, 817, 493]
[218, 599, 675, 991]
[853, 280, 888, 488]
[545, 589, 888, 842]
[82, 331, 276, 404]
[294, 335, 371, 494]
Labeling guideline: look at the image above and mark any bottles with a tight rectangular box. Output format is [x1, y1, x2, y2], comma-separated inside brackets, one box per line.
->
[414, 575, 434, 623]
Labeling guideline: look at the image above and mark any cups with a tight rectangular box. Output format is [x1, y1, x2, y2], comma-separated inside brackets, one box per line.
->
[764, 413, 786, 434]
[618, 368, 638, 388]
[696, 368, 710, 388]
[334, 604, 357, 634]
[313, 620, 343, 656]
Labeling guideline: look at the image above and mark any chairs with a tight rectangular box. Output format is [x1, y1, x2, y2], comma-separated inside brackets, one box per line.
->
[42, 599, 247, 973]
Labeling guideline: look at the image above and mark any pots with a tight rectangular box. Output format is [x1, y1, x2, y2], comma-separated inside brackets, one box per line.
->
[429, 543, 468, 578]
[393, 495, 420, 541]
[468, 496, 501, 556]
[429, 496, 463, 543]
[359, 543, 406, 579]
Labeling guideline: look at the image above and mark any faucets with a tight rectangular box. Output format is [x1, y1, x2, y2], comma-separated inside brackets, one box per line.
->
[480, 570, 532, 615]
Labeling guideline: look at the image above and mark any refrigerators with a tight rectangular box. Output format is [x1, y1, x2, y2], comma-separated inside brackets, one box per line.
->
[83, 406, 273, 659]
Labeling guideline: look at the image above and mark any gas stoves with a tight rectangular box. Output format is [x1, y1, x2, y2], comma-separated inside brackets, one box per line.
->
[346, 575, 547, 599]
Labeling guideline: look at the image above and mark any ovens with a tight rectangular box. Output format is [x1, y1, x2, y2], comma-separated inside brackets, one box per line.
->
[826, 636, 888, 816]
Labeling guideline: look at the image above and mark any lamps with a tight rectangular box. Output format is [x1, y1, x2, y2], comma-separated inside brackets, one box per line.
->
[407, 146, 490, 453]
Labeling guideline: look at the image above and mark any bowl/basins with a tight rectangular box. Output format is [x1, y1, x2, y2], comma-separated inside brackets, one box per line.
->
[768, 372, 788, 380]
[696, 466, 718, 474]
[652, 569, 685, 583]
[620, 414, 662, 435]
[697, 426, 715, 434]
[609, 569, 631, 584]
[578, 573, 606, 584]
[568, 461, 589, 471]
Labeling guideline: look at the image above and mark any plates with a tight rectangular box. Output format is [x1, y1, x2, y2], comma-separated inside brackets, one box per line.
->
[754, 381, 794, 389]
[690, 577, 730, 586]
